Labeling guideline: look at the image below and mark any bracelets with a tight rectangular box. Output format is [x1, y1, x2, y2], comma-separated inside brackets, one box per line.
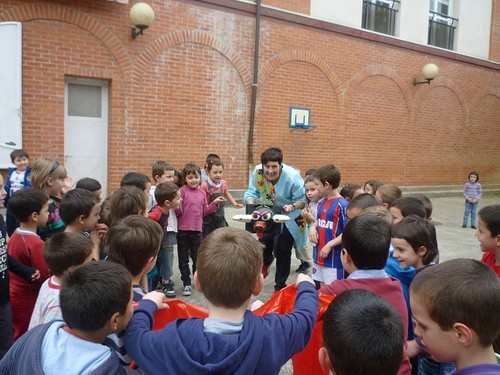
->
[291, 204, 295, 210]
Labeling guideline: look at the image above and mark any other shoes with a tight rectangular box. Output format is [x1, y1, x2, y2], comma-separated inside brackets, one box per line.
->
[274, 284, 287, 294]
[296, 262, 310, 273]
[462, 225, 467, 228]
[470, 225, 477, 229]
[261, 263, 269, 278]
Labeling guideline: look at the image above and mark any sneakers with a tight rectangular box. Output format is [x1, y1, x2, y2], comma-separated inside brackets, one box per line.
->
[182, 284, 192, 295]
[160, 279, 176, 298]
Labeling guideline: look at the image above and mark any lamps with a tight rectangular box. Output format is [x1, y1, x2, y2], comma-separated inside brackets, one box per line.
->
[413, 63, 438, 86]
[130, 2, 155, 40]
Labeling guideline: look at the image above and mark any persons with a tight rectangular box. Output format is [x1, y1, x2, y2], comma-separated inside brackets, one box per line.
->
[462, 171, 481, 229]
[0, 148, 500, 375]
